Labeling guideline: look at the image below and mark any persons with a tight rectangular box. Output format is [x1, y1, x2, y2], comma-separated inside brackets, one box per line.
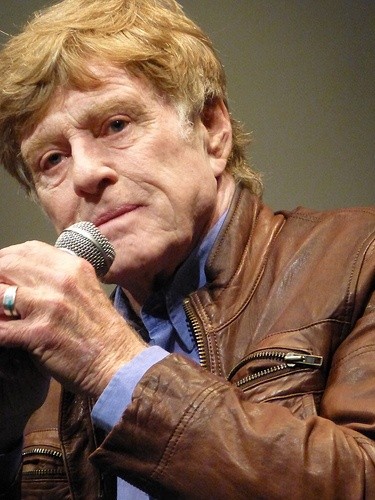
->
[0, 0, 375, 500]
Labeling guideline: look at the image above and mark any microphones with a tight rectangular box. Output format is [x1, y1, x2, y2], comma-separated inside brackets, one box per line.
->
[55, 221, 117, 281]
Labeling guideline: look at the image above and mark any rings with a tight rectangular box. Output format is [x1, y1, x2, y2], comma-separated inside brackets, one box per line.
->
[2, 285, 18, 316]
